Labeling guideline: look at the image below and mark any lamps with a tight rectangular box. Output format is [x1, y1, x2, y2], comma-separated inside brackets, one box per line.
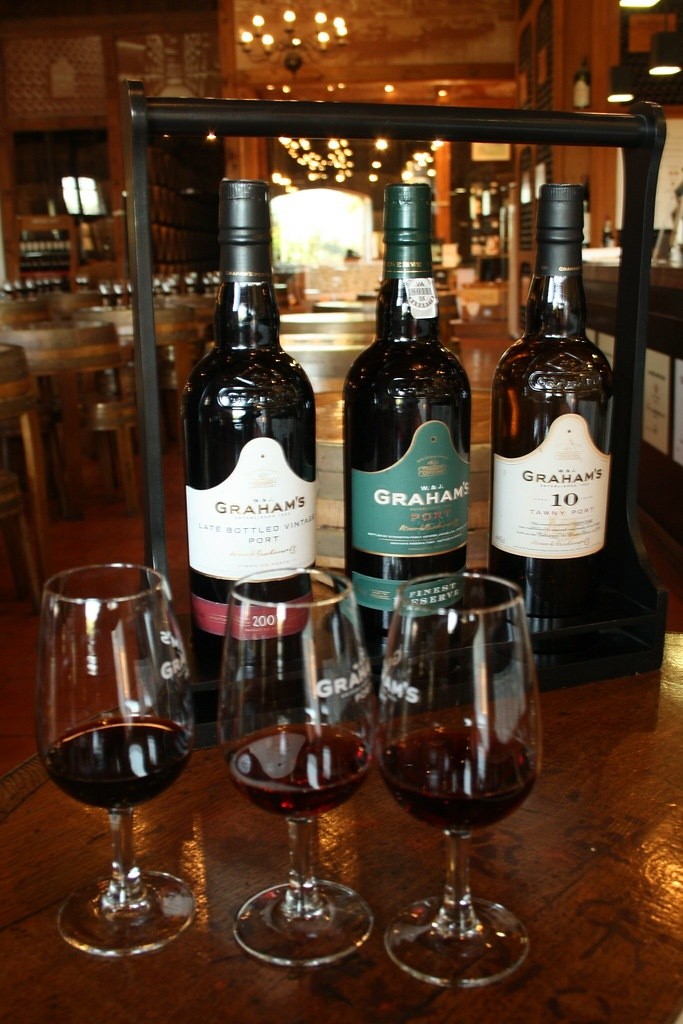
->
[649, 13, 681, 76]
[238, 8, 347, 79]
[608, 63, 633, 105]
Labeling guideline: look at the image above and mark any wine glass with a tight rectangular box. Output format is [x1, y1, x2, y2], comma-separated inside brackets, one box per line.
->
[376, 572, 543, 989]
[34, 563, 196, 957]
[214, 567, 374, 968]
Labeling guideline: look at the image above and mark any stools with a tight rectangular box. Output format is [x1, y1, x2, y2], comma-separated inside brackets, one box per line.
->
[0, 295, 215, 618]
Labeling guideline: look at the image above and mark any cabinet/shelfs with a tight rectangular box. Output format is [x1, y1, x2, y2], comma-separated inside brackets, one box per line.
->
[509, 1, 621, 338]
[144, 135, 221, 276]
[536, 265, 682, 545]
[14, 214, 124, 283]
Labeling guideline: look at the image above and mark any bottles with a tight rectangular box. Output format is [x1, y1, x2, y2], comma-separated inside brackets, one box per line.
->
[180, 177, 317, 726]
[488, 183, 615, 655]
[340, 184, 472, 694]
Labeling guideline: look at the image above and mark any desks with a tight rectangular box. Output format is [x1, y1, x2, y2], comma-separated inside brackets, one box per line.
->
[0, 629, 681, 1024]
